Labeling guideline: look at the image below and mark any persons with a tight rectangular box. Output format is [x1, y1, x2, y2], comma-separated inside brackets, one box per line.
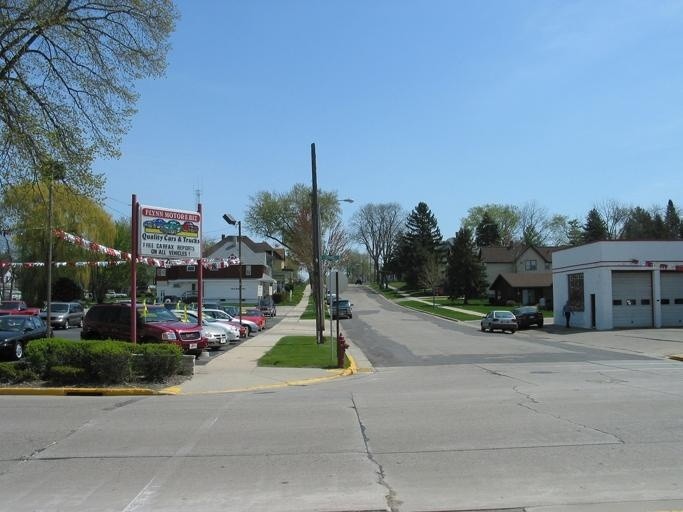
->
[561, 301, 574, 328]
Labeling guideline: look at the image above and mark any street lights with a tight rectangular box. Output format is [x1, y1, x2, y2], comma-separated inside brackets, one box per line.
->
[41, 160, 66, 337]
[223, 213, 242, 324]
[336, 198, 354, 203]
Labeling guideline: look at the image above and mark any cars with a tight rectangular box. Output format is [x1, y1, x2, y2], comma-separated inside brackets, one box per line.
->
[331, 300, 353, 319]
[0, 301, 276, 359]
[182, 292, 198, 304]
[481, 306, 544, 333]
